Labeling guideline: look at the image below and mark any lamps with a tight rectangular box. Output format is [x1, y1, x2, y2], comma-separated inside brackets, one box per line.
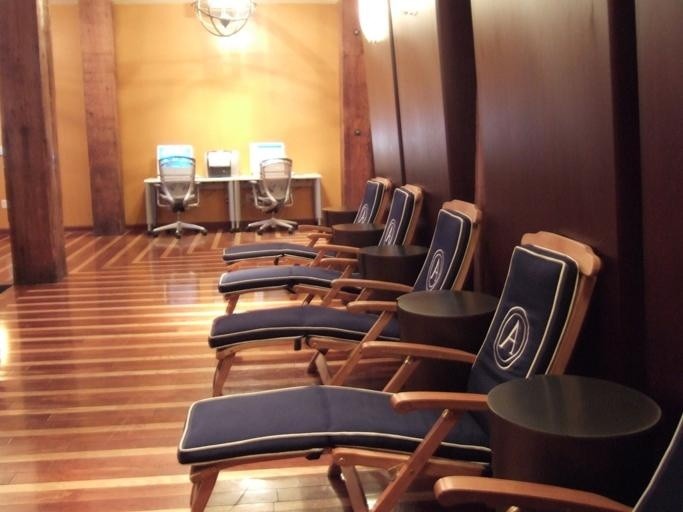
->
[191, 1, 257, 39]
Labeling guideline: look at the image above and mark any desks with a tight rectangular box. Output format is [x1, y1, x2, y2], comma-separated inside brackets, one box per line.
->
[143, 173, 322, 236]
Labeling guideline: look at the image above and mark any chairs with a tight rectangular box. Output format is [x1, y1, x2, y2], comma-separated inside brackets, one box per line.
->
[152, 154, 207, 239]
[208, 197, 485, 398]
[216, 183, 434, 314]
[222, 176, 397, 273]
[173, 229, 605, 512]
[245, 156, 299, 236]
[332, 373, 683, 512]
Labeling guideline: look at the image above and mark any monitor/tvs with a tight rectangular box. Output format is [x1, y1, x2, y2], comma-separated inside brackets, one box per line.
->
[156, 144, 194, 176]
[249, 143, 284, 177]
